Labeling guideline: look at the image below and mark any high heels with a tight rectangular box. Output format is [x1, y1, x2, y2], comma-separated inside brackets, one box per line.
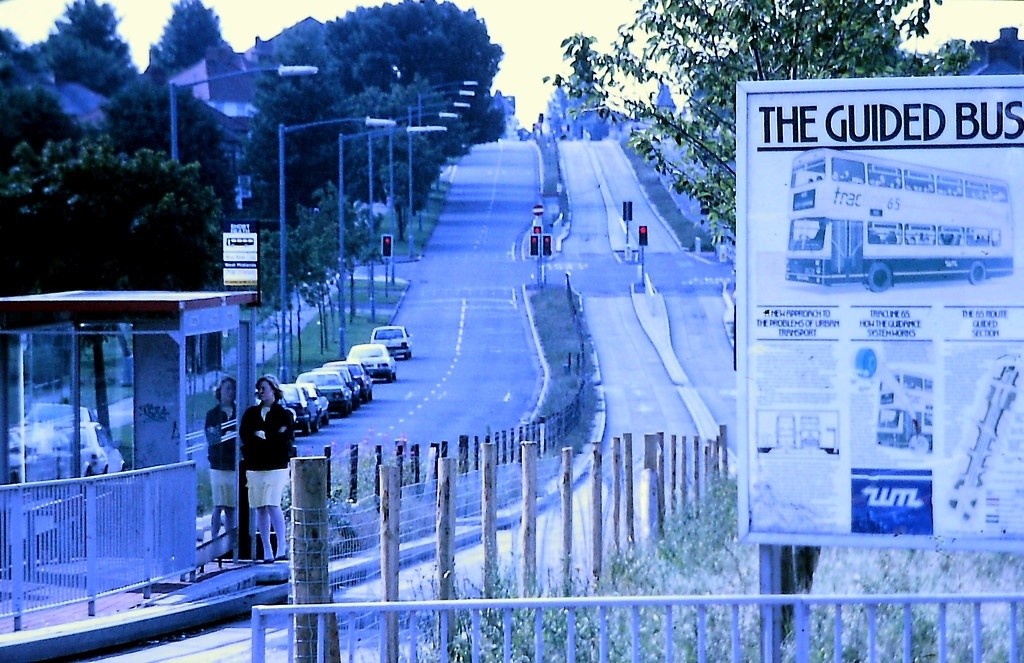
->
[263, 547, 277, 566]
[276, 540, 288, 562]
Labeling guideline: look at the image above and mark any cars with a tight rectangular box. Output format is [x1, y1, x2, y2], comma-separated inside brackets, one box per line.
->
[294, 372, 353, 418]
[370, 326, 412, 360]
[322, 361, 374, 403]
[311, 366, 361, 411]
[295, 383, 330, 427]
[8, 402, 127, 482]
[346, 344, 397, 383]
[279, 386, 321, 435]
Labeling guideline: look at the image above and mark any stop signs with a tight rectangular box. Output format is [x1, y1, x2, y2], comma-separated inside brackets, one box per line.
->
[532, 205, 543, 216]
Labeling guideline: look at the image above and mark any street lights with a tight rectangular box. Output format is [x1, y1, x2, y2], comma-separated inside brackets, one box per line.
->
[278, 117, 396, 379]
[338, 126, 448, 357]
[367, 111, 460, 248]
[409, 102, 470, 256]
[169, 64, 319, 162]
[417, 90, 475, 127]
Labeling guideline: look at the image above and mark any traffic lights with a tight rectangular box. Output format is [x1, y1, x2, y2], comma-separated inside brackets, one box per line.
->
[534, 226, 541, 234]
[383, 236, 391, 257]
[530, 235, 538, 256]
[639, 226, 648, 246]
[543, 236, 551, 256]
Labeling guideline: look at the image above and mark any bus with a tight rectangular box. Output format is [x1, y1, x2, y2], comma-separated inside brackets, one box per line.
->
[784, 148, 1013, 293]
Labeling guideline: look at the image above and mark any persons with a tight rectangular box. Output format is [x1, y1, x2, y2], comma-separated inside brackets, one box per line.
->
[238, 373, 295, 564]
[205, 375, 238, 540]
